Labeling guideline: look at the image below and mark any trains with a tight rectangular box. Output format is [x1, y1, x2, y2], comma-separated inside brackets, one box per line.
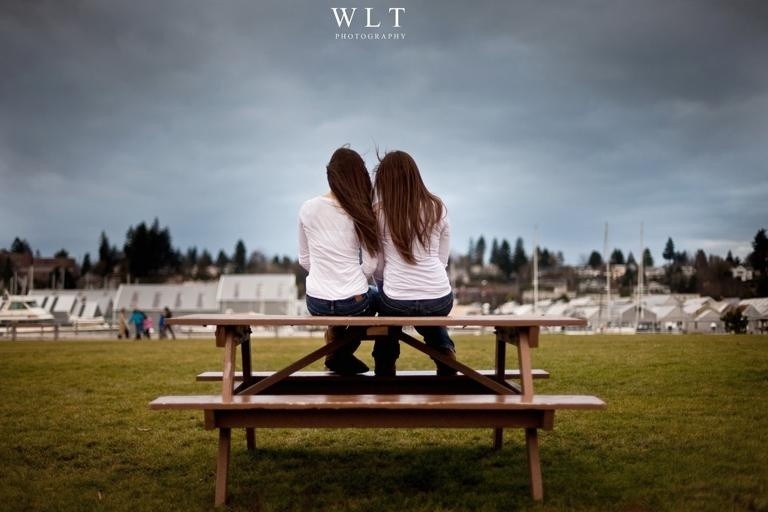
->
[0, 295, 55, 320]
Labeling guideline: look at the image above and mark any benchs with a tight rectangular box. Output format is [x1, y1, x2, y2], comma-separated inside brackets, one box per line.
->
[147, 394, 605, 506]
[196, 370, 551, 452]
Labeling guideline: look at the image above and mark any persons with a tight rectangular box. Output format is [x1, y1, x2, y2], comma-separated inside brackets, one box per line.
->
[157, 310, 166, 340]
[127, 306, 151, 339]
[295, 141, 382, 375]
[371, 149, 457, 374]
[116, 307, 130, 339]
[162, 305, 175, 340]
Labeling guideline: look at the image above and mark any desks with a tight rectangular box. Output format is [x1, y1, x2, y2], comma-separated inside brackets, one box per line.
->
[165, 308, 586, 508]
